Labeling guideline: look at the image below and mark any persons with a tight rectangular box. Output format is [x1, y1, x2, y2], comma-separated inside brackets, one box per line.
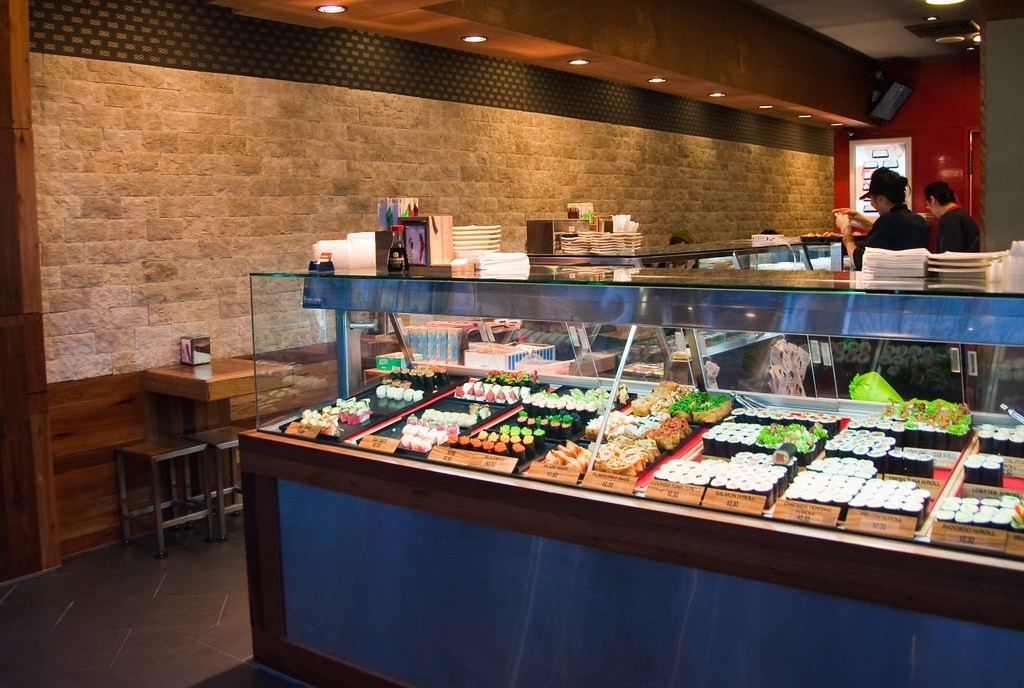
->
[656, 230, 699, 337]
[925, 181, 981, 271]
[832, 167, 931, 271]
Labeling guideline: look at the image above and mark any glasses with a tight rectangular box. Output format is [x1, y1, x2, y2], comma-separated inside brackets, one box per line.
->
[926, 200, 930, 209]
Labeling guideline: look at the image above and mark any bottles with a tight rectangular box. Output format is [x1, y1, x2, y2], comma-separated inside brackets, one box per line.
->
[387, 225, 409, 275]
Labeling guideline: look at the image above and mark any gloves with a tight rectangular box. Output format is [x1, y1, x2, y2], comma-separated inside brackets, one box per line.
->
[834, 212, 851, 234]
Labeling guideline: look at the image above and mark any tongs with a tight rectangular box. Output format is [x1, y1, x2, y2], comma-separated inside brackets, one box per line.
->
[1001, 404, 1024, 425]
[730, 390, 766, 409]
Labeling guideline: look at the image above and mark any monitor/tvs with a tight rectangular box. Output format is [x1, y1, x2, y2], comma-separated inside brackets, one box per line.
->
[867, 81, 913, 124]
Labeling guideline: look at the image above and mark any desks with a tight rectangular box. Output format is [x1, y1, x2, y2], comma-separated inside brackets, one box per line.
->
[142, 358, 296, 518]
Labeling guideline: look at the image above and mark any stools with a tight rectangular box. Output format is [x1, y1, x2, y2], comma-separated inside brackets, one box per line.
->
[115, 436, 217, 560]
[184, 426, 249, 543]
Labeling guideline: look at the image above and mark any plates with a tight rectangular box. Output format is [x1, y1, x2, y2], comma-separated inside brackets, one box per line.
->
[452, 224, 502, 257]
[927, 250, 1006, 272]
[562, 232, 641, 254]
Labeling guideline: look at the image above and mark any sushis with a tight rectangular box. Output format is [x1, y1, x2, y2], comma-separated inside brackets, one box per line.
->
[294, 362, 1024, 535]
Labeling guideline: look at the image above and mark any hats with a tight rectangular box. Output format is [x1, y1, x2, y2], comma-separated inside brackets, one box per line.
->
[859, 180, 907, 200]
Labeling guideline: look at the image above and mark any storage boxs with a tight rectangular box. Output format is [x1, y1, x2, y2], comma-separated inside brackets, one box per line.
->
[397, 215, 453, 265]
[465, 341, 556, 372]
[528, 218, 591, 255]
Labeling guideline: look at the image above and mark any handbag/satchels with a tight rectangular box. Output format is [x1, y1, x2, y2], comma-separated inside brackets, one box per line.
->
[849, 372, 903, 403]
